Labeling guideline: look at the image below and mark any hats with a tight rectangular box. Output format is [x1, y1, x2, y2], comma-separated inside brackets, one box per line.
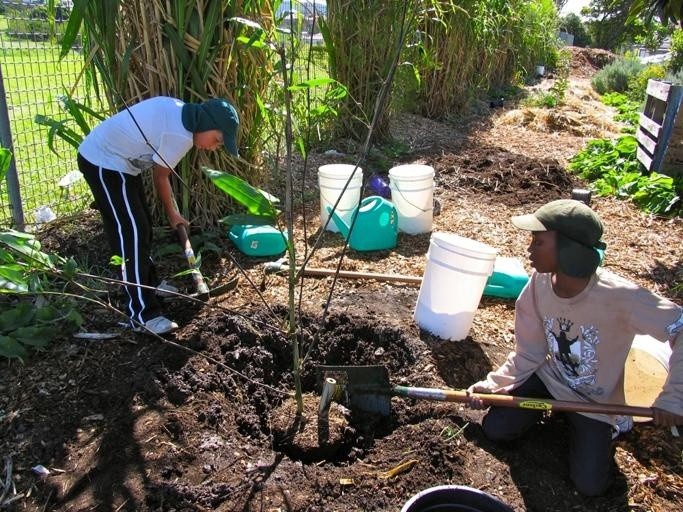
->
[509, 198, 606, 277]
[181, 97, 239, 156]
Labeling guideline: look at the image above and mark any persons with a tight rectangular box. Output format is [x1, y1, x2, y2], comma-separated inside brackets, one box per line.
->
[76, 97, 241, 335]
[464, 197, 683, 498]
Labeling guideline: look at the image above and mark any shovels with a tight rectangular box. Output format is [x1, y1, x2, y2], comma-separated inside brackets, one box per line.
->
[345, 365, 656, 420]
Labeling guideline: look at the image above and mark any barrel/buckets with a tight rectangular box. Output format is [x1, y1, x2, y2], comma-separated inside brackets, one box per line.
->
[560, 32, 574, 47]
[388, 161, 436, 236]
[413, 230, 496, 342]
[535, 64, 544, 75]
[317, 165, 363, 233]
[622, 332, 674, 424]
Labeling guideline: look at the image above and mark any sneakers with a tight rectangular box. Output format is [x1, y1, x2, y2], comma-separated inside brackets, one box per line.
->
[143, 317, 179, 334]
[154, 279, 179, 297]
